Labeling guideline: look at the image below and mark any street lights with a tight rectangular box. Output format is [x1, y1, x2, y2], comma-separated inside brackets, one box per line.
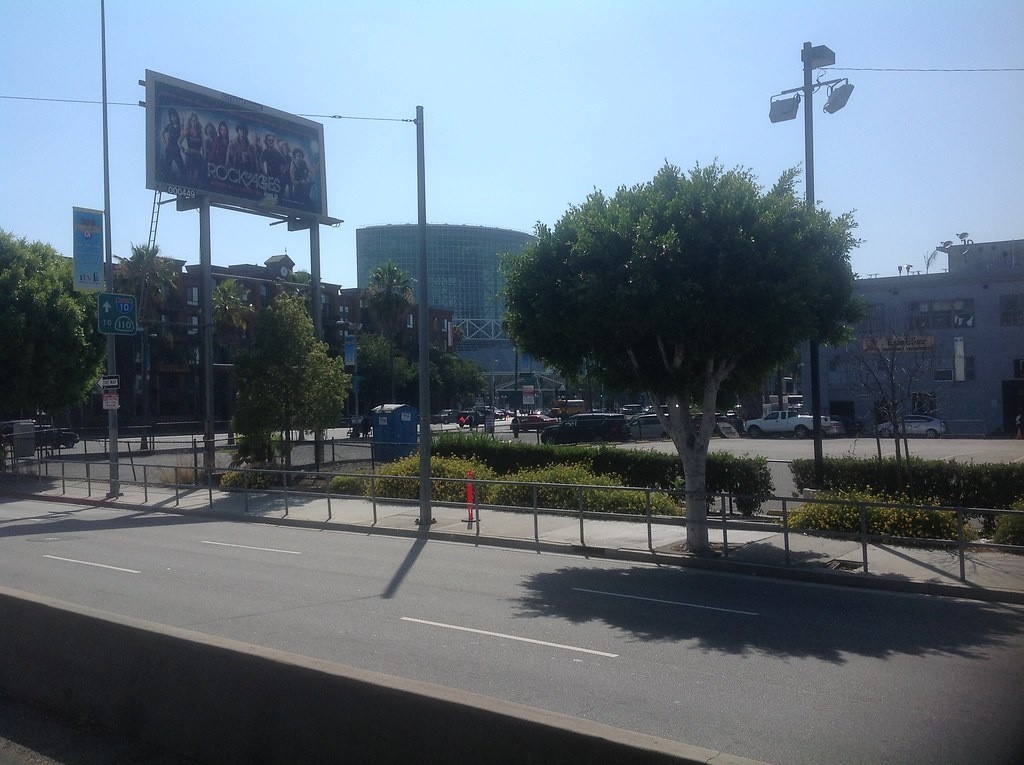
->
[769, 41, 854, 490]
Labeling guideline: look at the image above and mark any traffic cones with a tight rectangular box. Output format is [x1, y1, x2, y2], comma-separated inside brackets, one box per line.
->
[1015, 427, 1023, 439]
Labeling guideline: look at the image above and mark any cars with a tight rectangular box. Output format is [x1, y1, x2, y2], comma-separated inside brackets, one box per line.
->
[822, 415, 846, 438]
[623, 404, 669, 421]
[692, 413, 743, 434]
[341, 415, 364, 428]
[873, 415, 946, 439]
[431, 406, 515, 428]
[627, 414, 670, 439]
[34, 424, 79, 448]
[510, 417, 529, 432]
[522, 415, 556, 433]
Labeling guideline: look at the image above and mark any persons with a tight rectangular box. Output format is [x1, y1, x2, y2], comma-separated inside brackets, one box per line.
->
[163, 107, 313, 202]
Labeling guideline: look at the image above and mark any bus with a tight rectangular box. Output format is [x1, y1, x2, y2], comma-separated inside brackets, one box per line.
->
[556, 400, 586, 414]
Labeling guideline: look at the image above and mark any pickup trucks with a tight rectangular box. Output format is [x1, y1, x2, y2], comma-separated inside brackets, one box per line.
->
[744, 411, 830, 440]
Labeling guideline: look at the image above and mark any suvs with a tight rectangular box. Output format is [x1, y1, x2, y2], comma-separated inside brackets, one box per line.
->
[541, 413, 632, 444]
